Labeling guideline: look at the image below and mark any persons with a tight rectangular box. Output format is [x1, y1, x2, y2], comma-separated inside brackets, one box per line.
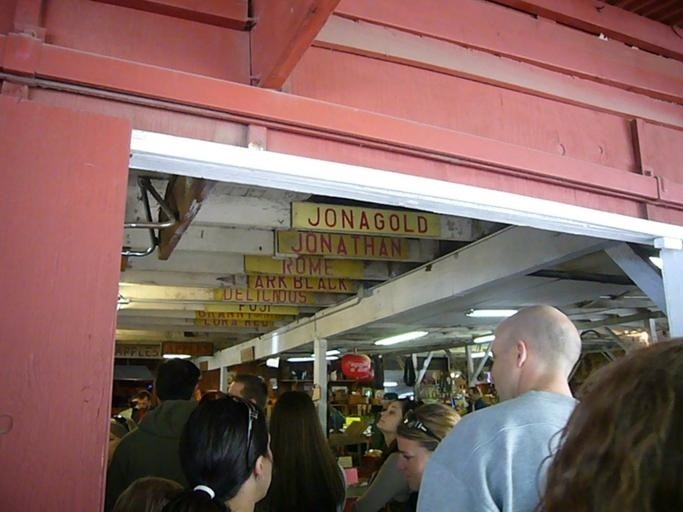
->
[106, 302, 682, 511]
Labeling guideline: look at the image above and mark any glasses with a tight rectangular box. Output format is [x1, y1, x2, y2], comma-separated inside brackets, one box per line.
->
[399, 413, 444, 447]
[197, 391, 261, 460]
